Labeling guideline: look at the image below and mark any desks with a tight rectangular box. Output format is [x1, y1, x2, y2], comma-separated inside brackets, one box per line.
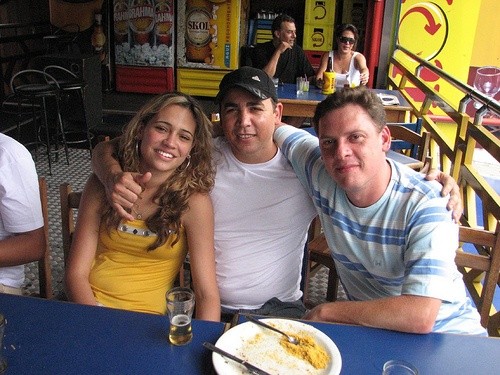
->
[276, 83, 413, 124]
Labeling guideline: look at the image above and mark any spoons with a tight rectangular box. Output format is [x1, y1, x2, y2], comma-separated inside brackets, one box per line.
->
[245, 314, 298, 345]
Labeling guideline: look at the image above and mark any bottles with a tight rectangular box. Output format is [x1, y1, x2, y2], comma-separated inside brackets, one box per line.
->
[185, 0, 213, 62]
[344, 75, 350, 88]
[114, 0, 172, 49]
[324, 57, 333, 72]
[91, 14, 106, 64]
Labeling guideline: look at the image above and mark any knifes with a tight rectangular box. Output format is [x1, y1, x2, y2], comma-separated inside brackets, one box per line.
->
[203, 342, 271, 375]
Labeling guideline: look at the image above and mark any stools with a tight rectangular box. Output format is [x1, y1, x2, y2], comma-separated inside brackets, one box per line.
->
[9, 69, 70, 175]
[43, 65, 94, 165]
[43, 23, 80, 60]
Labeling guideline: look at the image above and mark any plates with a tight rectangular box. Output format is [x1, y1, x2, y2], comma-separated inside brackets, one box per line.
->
[212, 319, 342, 375]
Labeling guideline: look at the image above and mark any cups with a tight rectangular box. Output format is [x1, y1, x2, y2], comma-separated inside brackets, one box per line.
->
[296, 77, 304, 95]
[165, 287, 194, 346]
[383, 359, 419, 375]
[322, 72, 336, 94]
[299, 81, 309, 92]
[271, 77, 279, 88]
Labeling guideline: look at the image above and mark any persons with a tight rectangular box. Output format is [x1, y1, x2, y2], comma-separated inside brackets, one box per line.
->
[211, 87, 490, 336]
[250, 14, 316, 125]
[92, 66, 465, 322]
[0, 132, 46, 297]
[67, 92, 221, 323]
[317, 23, 370, 89]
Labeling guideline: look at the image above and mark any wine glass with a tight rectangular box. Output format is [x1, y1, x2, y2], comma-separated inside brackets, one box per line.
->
[472, 67, 500, 118]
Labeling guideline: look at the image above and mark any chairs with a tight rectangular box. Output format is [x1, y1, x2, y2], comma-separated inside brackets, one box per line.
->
[388, 123, 431, 171]
[300, 155, 434, 309]
[454, 216, 500, 329]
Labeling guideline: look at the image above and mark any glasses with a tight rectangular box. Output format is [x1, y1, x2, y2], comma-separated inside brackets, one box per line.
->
[339, 36, 356, 44]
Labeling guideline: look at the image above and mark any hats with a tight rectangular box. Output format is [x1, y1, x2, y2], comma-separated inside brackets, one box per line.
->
[215, 66, 277, 105]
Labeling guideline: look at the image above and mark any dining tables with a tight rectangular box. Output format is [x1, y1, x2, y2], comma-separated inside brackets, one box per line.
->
[0, 294, 500, 375]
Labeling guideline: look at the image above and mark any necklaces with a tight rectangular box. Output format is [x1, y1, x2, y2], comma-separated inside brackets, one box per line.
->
[132, 206, 155, 219]
